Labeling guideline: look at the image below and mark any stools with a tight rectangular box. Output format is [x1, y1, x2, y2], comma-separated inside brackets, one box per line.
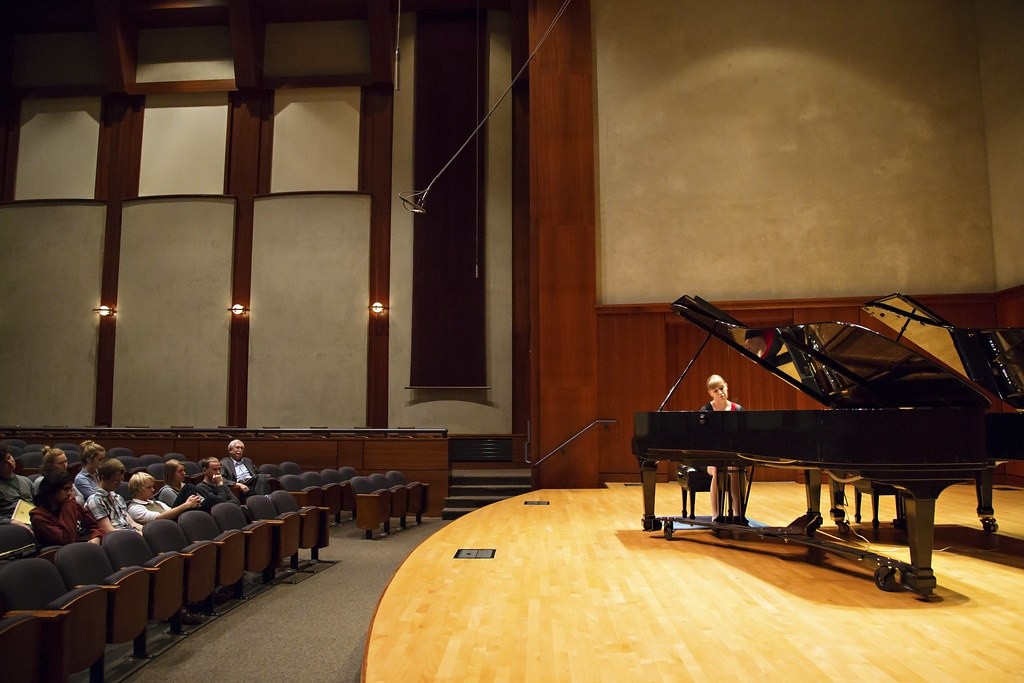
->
[677, 463, 747, 521]
[854, 479, 907, 529]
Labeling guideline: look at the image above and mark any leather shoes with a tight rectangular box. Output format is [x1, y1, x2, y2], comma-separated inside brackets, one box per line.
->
[181, 608, 203, 624]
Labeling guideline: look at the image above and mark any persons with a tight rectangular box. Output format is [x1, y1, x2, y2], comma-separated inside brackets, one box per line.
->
[219, 439, 282, 504]
[0, 448, 38, 538]
[34, 445, 85, 505]
[698, 374, 750, 525]
[195, 455, 242, 516]
[157, 458, 186, 508]
[74, 440, 105, 500]
[83, 458, 144, 536]
[125, 471, 202, 525]
[29, 469, 105, 546]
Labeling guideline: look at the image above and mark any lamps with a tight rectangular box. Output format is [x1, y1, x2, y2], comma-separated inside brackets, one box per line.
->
[93, 305, 117, 316]
[227, 303, 250, 314]
[368, 302, 389, 313]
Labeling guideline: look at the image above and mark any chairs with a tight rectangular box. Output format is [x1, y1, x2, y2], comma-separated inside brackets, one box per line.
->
[0, 438, 433, 683]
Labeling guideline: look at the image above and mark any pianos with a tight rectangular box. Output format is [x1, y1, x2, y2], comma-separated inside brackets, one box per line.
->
[860, 290, 1024, 533]
[630, 294, 992, 599]
